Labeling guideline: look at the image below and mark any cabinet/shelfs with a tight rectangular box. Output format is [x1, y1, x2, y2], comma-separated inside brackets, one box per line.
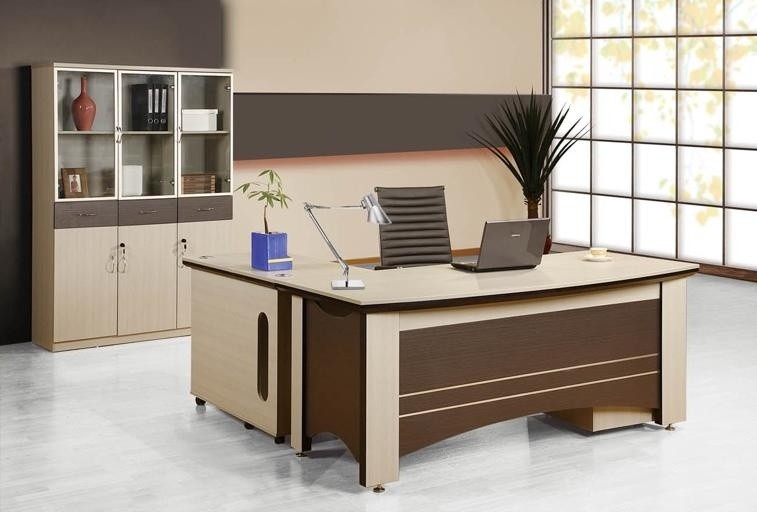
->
[29, 60, 235, 354]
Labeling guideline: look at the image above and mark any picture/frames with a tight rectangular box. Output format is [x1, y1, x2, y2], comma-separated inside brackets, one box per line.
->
[61, 167, 89, 198]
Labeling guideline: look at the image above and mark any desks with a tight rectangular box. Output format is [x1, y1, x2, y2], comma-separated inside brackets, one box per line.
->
[181, 251, 701, 494]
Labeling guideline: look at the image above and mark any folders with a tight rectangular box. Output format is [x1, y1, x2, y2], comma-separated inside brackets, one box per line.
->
[131, 84, 168, 131]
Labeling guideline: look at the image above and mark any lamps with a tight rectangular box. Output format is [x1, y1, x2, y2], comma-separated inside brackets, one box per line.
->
[303, 194, 393, 290]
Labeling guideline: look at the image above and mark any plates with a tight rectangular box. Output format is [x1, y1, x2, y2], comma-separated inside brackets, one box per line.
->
[583, 254, 614, 263]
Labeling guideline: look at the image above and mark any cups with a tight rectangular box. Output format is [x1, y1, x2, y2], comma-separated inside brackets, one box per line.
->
[590, 247, 608, 256]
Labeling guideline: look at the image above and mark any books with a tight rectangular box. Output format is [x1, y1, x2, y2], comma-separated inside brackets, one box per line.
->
[152, 83, 162, 130]
[159, 83, 169, 131]
[131, 84, 153, 130]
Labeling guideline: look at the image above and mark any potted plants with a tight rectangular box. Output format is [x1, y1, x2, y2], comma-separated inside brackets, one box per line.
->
[234, 168, 293, 272]
[465, 83, 595, 253]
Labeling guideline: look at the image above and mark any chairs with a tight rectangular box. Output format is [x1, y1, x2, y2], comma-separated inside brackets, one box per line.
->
[374, 186, 454, 270]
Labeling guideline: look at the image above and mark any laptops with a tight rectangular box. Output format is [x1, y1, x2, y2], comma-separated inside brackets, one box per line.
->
[450, 218, 551, 273]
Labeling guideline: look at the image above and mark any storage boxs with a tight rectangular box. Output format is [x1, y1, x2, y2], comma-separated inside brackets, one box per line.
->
[181, 174, 216, 194]
[181, 108, 218, 131]
